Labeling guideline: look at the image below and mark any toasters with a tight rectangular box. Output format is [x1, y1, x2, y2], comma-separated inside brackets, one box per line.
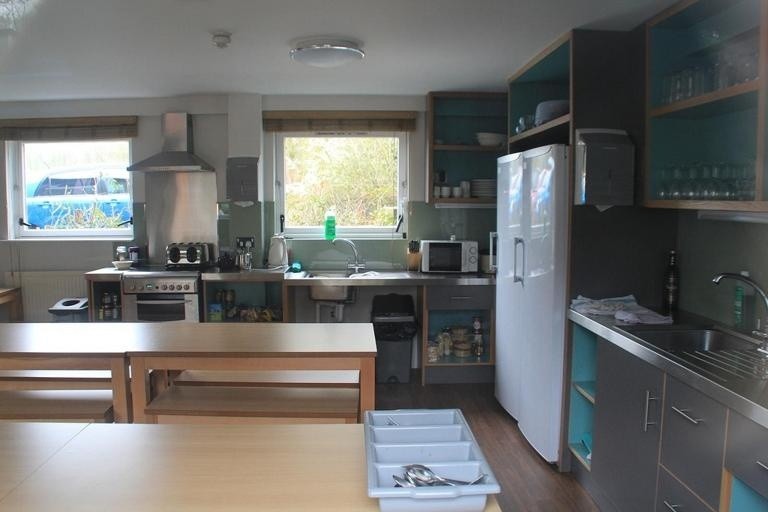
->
[165, 241, 214, 266]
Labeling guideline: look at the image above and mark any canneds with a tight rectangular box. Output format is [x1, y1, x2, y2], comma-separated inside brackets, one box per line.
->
[128, 247, 140, 266]
[116, 245, 128, 261]
[473, 315, 484, 357]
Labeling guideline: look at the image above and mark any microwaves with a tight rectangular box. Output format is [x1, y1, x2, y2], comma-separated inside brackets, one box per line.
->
[420, 239, 479, 274]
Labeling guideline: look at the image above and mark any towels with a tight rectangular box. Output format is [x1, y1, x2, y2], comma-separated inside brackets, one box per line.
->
[569, 294, 636, 316]
[614, 310, 674, 324]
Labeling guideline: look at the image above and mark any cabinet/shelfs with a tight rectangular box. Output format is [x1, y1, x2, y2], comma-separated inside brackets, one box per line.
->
[564, 320, 667, 512]
[641, 1, 768, 212]
[424, 91, 510, 204]
[723, 406, 768, 499]
[417, 285, 497, 386]
[507, 27, 642, 206]
[656, 372, 768, 512]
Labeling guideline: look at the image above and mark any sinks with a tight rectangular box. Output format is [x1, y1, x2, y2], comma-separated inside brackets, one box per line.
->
[305, 270, 350, 279]
[625, 328, 760, 353]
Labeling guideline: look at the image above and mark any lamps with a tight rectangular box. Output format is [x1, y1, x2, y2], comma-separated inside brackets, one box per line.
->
[290, 38, 366, 69]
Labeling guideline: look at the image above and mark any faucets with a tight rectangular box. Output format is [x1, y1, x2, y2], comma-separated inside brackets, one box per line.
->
[713, 272, 768, 353]
[328, 238, 365, 273]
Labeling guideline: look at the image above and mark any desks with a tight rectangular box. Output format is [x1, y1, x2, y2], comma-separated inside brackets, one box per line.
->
[0, 420, 501, 512]
[1, 288, 23, 322]
[126, 323, 377, 423]
[0, 323, 133, 424]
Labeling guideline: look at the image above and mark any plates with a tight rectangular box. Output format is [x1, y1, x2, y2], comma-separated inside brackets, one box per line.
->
[535, 99, 569, 125]
[473, 178, 498, 197]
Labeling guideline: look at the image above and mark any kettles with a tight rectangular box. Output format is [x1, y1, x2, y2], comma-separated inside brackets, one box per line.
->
[267, 236, 288, 267]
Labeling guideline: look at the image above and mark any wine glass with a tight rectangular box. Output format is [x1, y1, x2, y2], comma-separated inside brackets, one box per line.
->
[656, 162, 757, 199]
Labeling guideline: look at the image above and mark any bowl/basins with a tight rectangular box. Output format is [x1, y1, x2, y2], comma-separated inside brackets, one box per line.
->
[476, 132, 505, 145]
[434, 181, 471, 198]
[112, 261, 134, 270]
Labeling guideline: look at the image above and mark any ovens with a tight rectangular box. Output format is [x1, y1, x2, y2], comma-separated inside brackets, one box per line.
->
[124, 277, 201, 322]
[489, 146, 576, 471]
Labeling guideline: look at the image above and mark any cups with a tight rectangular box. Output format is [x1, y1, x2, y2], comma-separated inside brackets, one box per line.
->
[657, 69, 704, 103]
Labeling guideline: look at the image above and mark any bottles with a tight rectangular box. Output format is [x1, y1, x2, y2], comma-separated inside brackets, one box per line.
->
[662, 249, 679, 310]
[100, 290, 120, 320]
[733, 269, 752, 330]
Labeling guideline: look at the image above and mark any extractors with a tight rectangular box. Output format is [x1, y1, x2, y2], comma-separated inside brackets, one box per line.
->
[126, 112, 216, 173]
